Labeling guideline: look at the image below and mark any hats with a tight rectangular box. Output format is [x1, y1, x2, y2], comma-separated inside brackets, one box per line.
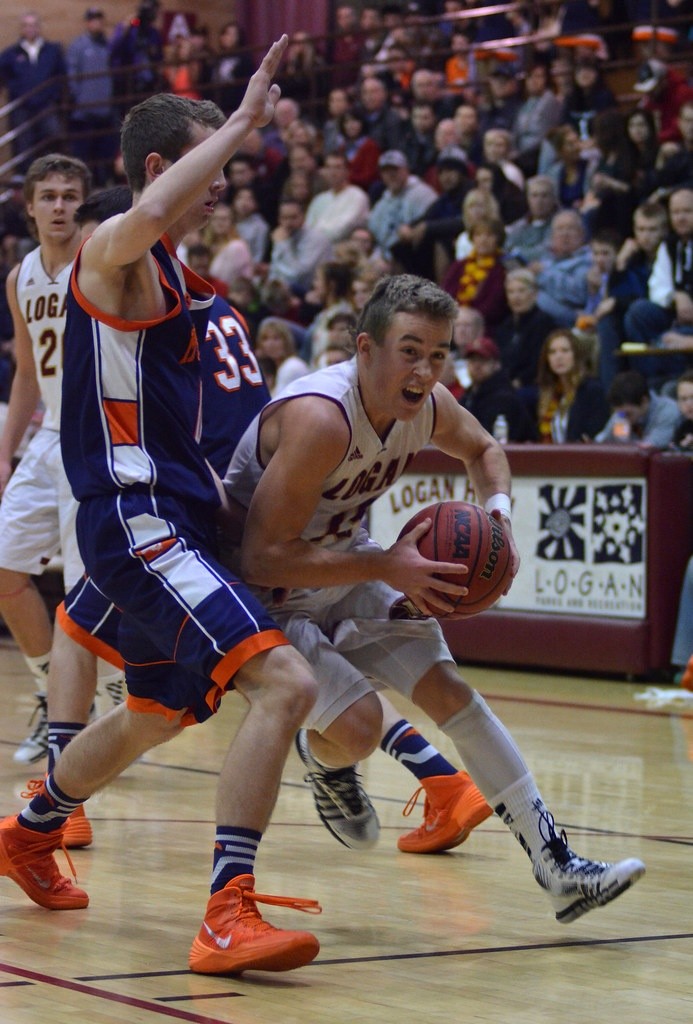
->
[375, 149, 407, 172]
[437, 143, 469, 173]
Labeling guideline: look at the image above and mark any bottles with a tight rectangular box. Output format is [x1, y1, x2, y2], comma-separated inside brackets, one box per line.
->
[612, 411, 630, 443]
[492, 413, 509, 446]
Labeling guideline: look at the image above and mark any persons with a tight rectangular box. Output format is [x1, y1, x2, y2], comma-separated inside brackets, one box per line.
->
[0, 34, 323, 974]
[17, 184, 493, 854]
[224, 274, 646, 925]
[0, 153, 127, 754]
[0, 0, 693, 449]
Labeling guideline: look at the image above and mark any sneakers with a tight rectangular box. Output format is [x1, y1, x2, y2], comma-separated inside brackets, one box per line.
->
[397, 770, 494, 855]
[186, 874, 323, 976]
[295, 728, 382, 853]
[1, 814, 91, 910]
[20, 770, 93, 848]
[531, 801, 647, 926]
[11, 690, 98, 767]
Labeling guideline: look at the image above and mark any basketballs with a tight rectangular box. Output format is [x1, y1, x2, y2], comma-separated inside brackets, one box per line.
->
[396, 501, 514, 621]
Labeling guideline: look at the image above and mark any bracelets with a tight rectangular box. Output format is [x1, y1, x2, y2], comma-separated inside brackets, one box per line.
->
[484, 493, 512, 521]
